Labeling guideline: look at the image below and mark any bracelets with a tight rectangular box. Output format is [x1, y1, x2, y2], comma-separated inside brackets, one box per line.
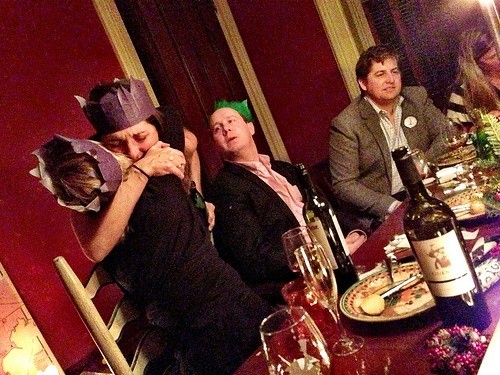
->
[132, 164, 149, 179]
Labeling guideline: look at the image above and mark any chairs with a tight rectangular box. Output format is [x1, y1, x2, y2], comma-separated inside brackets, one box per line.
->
[309, 159, 385, 216]
[52, 253, 178, 375]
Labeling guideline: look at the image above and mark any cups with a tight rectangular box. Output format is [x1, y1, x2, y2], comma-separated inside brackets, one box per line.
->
[282, 280, 317, 306]
[282, 226, 320, 279]
[260, 306, 331, 375]
[408, 148, 431, 179]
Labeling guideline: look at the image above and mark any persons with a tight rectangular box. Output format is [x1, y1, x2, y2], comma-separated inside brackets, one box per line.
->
[329, 28, 500, 241]
[28, 75, 281, 375]
[202, 99, 330, 303]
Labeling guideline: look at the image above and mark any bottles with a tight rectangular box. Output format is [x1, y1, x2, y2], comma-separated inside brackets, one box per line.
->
[294, 162, 361, 295]
[391, 145, 492, 331]
[469, 106, 495, 161]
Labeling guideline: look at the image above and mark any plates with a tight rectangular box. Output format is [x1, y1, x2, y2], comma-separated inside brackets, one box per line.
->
[339, 260, 437, 323]
[442, 189, 486, 224]
[427, 146, 477, 166]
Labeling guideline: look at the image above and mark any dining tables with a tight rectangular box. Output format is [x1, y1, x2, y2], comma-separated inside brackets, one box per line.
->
[233, 167, 500, 375]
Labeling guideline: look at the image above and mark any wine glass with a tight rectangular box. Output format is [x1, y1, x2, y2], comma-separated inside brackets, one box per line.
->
[439, 117, 472, 171]
[294, 242, 365, 357]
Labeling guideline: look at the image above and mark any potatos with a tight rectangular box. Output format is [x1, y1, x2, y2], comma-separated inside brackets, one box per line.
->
[471, 202, 486, 214]
[361, 293, 384, 315]
